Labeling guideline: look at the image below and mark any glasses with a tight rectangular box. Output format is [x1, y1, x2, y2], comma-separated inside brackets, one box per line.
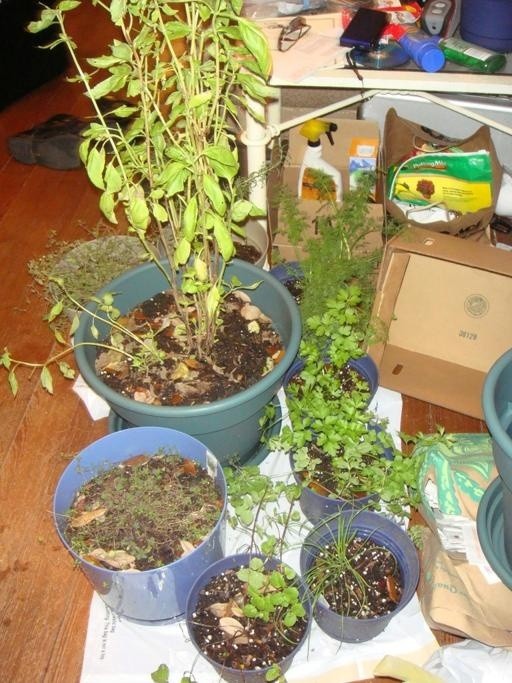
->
[276, 13, 312, 52]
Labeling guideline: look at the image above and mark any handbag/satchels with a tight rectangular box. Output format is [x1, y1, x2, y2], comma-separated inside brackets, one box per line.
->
[381, 104, 505, 246]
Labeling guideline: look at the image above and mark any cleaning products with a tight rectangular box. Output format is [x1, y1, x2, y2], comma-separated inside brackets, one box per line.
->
[297, 118, 342, 205]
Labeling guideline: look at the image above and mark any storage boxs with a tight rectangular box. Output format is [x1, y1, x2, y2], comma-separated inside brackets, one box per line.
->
[366, 229, 512, 422]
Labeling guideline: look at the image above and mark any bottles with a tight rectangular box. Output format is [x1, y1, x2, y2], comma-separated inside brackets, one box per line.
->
[399, 31, 507, 74]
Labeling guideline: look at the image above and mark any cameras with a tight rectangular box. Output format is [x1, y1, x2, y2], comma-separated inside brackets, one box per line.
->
[340, 7, 388, 50]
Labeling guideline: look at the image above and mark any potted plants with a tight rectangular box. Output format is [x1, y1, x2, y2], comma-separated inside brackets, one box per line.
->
[300, 508, 420, 645]
[0, 0, 302, 464]
[54, 427, 228, 623]
[184, 477, 314, 680]
[271, 174, 397, 517]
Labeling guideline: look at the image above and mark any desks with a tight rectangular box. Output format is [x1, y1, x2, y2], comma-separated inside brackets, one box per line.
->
[243, 13, 512, 273]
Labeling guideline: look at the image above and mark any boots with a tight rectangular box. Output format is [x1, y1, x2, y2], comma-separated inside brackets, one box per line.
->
[8, 93, 160, 169]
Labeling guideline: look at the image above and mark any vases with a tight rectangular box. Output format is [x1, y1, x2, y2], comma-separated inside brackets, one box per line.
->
[476, 346, 512, 593]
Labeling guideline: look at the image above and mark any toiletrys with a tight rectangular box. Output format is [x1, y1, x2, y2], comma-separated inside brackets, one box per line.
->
[394, 20, 446, 73]
[429, 33, 507, 75]
